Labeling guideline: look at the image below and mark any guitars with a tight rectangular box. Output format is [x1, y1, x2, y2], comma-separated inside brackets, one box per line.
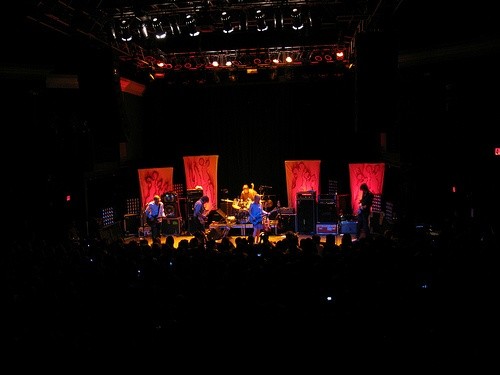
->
[198, 204, 215, 225]
[146, 216, 165, 226]
[249, 207, 277, 225]
[357, 205, 366, 214]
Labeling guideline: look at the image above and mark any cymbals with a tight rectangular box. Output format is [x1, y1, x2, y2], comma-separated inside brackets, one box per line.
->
[221, 199, 233, 203]
[265, 195, 276, 196]
[259, 185, 272, 189]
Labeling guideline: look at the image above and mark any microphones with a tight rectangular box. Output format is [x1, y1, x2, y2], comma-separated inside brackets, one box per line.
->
[144, 203, 148, 207]
[335, 191, 338, 196]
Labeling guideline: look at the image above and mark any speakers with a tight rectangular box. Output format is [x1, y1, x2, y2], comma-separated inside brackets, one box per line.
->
[187, 189, 203, 217]
[318, 194, 337, 224]
[124, 214, 142, 237]
[161, 192, 178, 203]
[213, 209, 226, 222]
[338, 195, 353, 219]
[296, 191, 317, 234]
[370, 211, 383, 233]
[162, 203, 179, 218]
[161, 218, 181, 236]
[275, 214, 296, 235]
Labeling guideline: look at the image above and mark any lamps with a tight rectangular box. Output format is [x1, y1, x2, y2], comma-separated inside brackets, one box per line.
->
[137, 45, 355, 70]
[110, 0, 313, 41]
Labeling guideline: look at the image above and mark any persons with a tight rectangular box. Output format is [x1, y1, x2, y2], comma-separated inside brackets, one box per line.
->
[39, 231, 426, 285]
[240, 184, 258, 202]
[249, 195, 271, 243]
[145, 195, 168, 244]
[193, 196, 210, 233]
[355, 184, 375, 241]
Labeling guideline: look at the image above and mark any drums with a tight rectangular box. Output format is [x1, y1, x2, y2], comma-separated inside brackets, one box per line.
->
[246, 198, 253, 208]
[260, 200, 266, 206]
[239, 202, 247, 207]
[232, 198, 244, 210]
[237, 208, 250, 224]
